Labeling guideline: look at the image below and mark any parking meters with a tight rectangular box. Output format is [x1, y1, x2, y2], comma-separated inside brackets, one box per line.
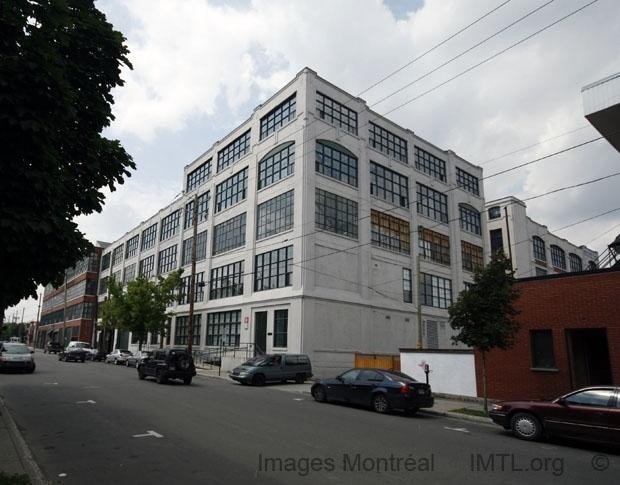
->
[424, 364, 430, 384]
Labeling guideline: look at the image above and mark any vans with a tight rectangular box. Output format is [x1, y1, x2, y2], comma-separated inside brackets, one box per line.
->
[228, 352, 314, 387]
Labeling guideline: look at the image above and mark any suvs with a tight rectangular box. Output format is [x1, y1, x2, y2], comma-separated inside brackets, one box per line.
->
[136, 348, 197, 386]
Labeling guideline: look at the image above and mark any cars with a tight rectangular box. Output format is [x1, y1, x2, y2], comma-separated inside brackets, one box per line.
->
[0, 336, 36, 373]
[44, 341, 150, 370]
[487, 384, 620, 453]
[310, 367, 435, 415]
